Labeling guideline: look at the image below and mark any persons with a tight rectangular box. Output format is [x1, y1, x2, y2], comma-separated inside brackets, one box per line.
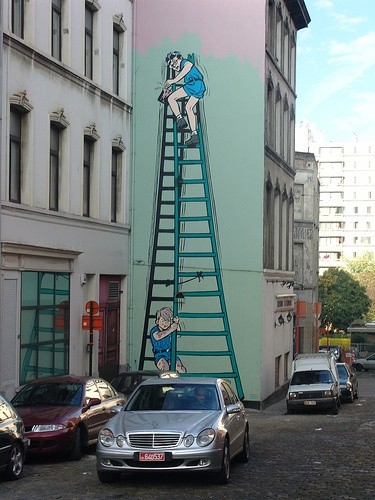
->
[189, 388, 211, 409]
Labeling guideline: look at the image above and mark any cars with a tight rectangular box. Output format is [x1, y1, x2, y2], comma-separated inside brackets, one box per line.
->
[352, 352, 375, 371]
[335, 363, 359, 403]
[0, 370, 181, 482]
[96, 377, 250, 485]
[286, 370, 341, 414]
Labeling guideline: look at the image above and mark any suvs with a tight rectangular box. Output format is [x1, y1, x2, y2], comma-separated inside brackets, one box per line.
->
[290, 353, 339, 380]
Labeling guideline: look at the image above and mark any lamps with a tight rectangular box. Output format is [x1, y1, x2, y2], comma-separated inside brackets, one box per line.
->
[275, 312, 292, 327]
